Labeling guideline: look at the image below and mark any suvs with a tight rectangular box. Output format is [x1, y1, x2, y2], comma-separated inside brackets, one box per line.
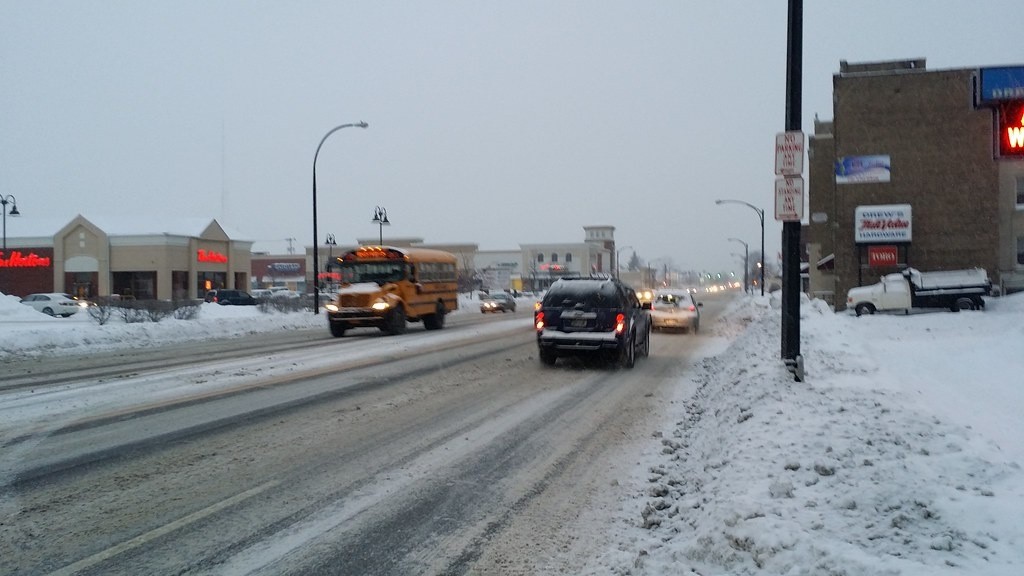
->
[205, 289, 260, 306]
[535, 274, 652, 368]
[252, 287, 339, 302]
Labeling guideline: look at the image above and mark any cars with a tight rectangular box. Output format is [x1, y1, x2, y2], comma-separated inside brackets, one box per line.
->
[19, 293, 79, 318]
[651, 289, 704, 333]
[479, 293, 517, 314]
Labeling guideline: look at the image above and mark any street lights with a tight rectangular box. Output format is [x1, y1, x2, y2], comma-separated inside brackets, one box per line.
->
[313, 121, 369, 313]
[716, 199, 765, 297]
[370, 206, 390, 246]
[325, 232, 339, 264]
[0, 193, 20, 252]
[615, 246, 633, 281]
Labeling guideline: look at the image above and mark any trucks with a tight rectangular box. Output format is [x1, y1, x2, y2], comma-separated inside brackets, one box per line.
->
[848, 265, 993, 316]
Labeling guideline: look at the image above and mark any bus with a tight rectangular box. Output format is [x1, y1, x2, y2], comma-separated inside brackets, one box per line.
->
[327, 245, 460, 337]
